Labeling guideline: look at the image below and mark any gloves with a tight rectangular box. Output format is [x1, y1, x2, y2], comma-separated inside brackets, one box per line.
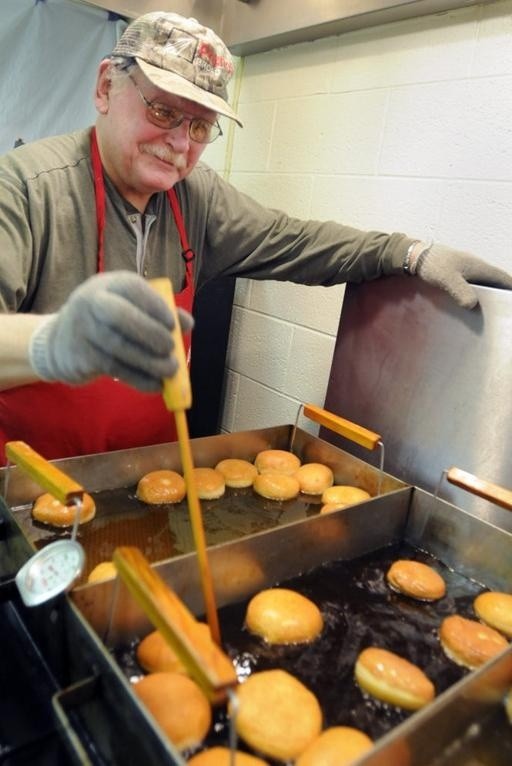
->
[27, 268, 196, 398]
[404, 238, 512, 313]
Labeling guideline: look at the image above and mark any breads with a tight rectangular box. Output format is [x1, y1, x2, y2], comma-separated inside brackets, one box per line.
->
[294, 725, 373, 766]
[189, 746, 270, 766]
[356, 647, 437, 708]
[440, 614, 508, 671]
[134, 469, 186, 504]
[253, 450, 335, 501]
[245, 588, 325, 646]
[473, 591, 511, 641]
[137, 622, 213, 677]
[386, 558, 446, 600]
[134, 672, 211, 752]
[227, 667, 323, 761]
[214, 459, 257, 487]
[87, 561, 119, 584]
[320, 486, 372, 515]
[31, 491, 95, 528]
[194, 467, 225, 500]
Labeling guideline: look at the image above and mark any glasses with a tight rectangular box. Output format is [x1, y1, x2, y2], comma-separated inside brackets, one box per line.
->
[112, 56, 227, 146]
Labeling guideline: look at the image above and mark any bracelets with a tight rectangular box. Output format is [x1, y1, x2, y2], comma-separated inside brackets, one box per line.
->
[403, 239, 419, 274]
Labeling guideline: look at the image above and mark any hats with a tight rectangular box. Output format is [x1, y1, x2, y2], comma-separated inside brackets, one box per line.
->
[110, 6, 248, 131]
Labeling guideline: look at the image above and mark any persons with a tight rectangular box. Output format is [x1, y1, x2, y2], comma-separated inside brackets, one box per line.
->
[0, 9, 511, 466]
